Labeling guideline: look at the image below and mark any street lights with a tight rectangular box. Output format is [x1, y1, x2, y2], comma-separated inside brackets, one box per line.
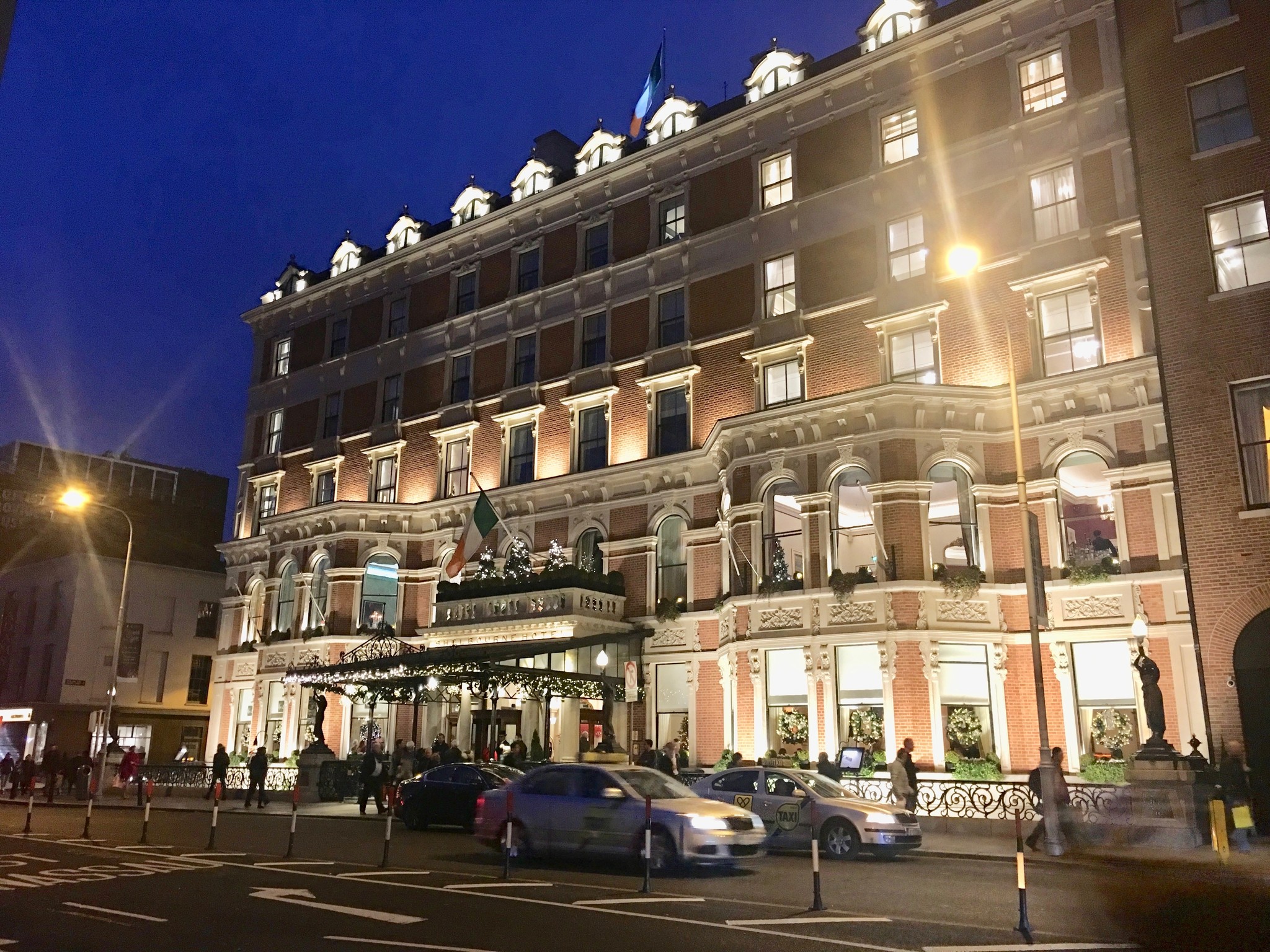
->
[1129, 610, 1186, 760]
[594, 649, 627, 754]
[62, 489, 134, 800]
[945, 242, 1066, 858]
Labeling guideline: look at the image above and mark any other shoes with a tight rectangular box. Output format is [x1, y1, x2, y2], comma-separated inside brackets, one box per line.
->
[1025, 842, 1040, 851]
[258, 805, 264, 809]
[360, 811, 366, 814]
[244, 803, 251, 806]
[378, 809, 387, 814]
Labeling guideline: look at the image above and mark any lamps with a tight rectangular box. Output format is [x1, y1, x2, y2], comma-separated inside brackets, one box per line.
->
[252, 730, 270, 751]
[1130, 612, 1151, 654]
[848, 714, 862, 749]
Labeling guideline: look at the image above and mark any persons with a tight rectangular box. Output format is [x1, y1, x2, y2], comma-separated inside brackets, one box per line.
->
[601, 734, 614, 753]
[1090, 530, 1118, 557]
[390, 733, 462, 785]
[205, 744, 230, 800]
[503, 734, 527, 770]
[636, 739, 657, 770]
[313, 691, 327, 745]
[728, 753, 742, 769]
[661, 739, 680, 782]
[119, 746, 139, 799]
[1025, 747, 1080, 851]
[358, 744, 387, 814]
[816, 752, 842, 783]
[1134, 651, 1166, 739]
[244, 747, 270, 808]
[1215, 741, 1251, 855]
[0, 745, 92, 800]
[891, 749, 913, 810]
[580, 731, 590, 753]
[903, 738, 918, 814]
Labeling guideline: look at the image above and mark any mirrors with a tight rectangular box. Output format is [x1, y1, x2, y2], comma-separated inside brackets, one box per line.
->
[941, 536, 972, 570]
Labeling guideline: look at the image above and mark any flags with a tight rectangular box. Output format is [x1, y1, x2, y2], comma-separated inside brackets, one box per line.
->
[630, 41, 664, 138]
[446, 491, 498, 578]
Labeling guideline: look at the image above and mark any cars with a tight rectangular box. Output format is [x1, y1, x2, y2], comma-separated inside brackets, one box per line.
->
[685, 758, 922, 860]
[473, 762, 768, 878]
[393, 760, 527, 834]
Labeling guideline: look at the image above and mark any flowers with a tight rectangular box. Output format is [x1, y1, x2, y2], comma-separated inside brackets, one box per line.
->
[272, 721, 283, 744]
[1092, 707, 1134, 750]
[712, 750, 743, 772]
[776, 752, 797, 759]
[302, 722, 321, 745]
[943, 706, 983, 747]
[849, 710, 880, 743]
[775, 711, 811, 744]
[240, 727, 252, 748]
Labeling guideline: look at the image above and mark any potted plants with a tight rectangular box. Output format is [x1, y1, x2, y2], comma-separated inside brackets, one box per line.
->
[837, 750, 887, 776]
[943, 750, 1003, 782]
[762, 749, 813, 767]
